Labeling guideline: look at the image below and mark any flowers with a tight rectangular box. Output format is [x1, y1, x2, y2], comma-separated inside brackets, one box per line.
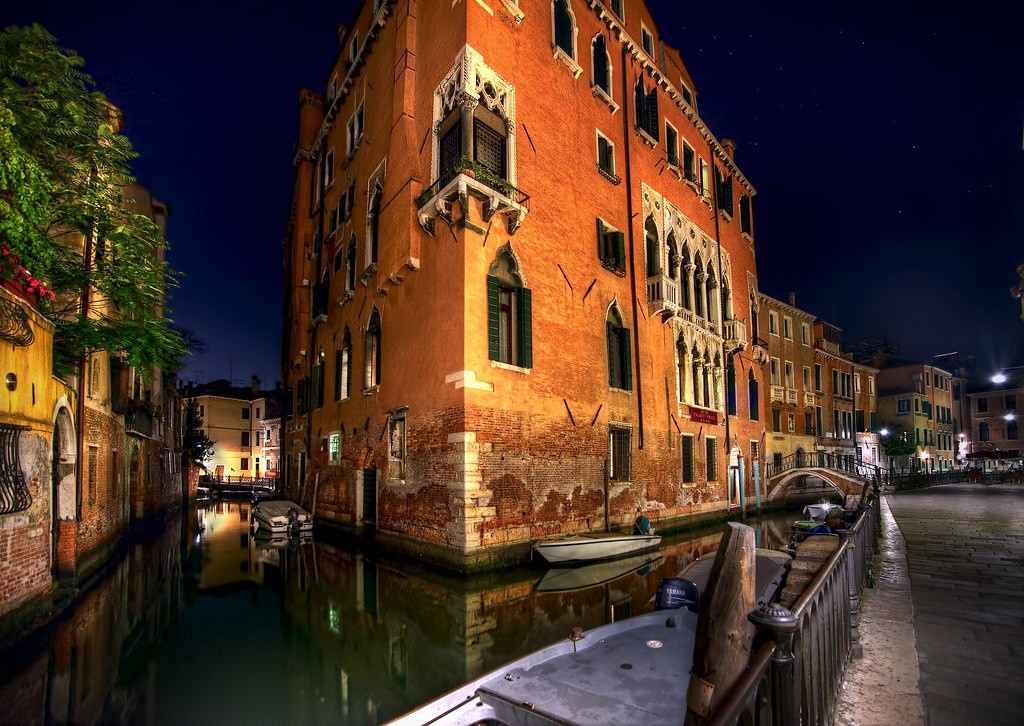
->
[0, 247, 57, 300]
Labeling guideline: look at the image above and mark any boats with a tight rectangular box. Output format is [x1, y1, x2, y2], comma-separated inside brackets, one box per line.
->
[254, 528, 315, 548]
[655, 504, 857, 620]
[381, 605, 698, 726]
[533, 533, 665, 564]
[533, 552, 664, 595]
[251, 487, 273, 501]
[252, 500, 314, 533]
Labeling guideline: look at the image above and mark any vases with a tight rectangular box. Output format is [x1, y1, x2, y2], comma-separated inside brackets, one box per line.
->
[0, 278, 36, 309]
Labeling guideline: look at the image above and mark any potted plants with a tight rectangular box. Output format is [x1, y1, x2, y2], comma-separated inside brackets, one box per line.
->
[456, 155, 482, 179]
